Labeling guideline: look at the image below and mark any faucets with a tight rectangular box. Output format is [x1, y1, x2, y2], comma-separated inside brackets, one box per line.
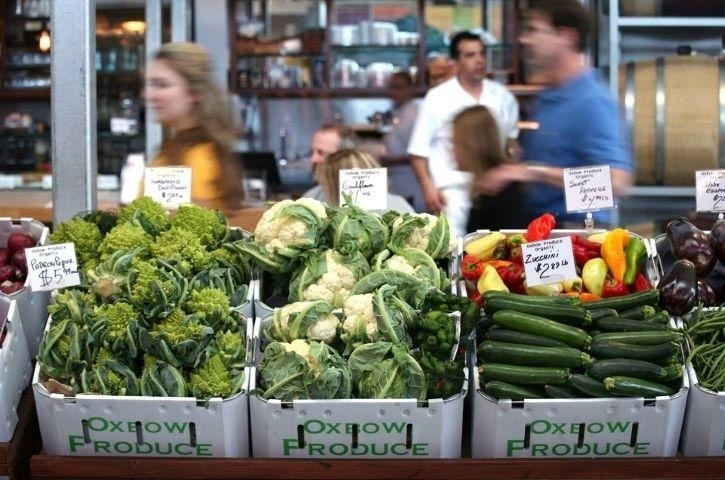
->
[278, 126, 287, 168]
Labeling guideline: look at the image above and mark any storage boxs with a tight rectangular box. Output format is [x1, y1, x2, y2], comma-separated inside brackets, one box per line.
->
[31, 220, 252, 458]
[0, 291, 30, 442]
[654, 231, 724, 456]
[0, 216, 53, 358]
[249, 228, 472, 461]
[457, 228, 692, 459]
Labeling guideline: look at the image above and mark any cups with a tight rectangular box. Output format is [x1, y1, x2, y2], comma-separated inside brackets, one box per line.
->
[234, 12, 425, 95]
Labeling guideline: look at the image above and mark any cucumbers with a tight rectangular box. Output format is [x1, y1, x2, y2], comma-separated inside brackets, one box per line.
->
[476, 290, 685, 406]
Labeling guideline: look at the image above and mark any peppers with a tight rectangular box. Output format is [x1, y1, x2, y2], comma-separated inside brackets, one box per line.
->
[461, 212, 652, 307]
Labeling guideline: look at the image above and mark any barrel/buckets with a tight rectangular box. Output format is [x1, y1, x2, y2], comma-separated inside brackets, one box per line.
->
[603, 53, 725, 187]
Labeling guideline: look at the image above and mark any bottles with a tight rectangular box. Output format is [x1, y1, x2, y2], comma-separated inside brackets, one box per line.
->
[2, 1, 151, 191]
[116, 152, 145, 206]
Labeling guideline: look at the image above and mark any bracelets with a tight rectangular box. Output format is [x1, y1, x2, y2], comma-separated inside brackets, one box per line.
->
[527, 163, 546, 183]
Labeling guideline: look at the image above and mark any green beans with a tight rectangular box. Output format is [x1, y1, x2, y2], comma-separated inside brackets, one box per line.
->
[679, 300, 725, 391]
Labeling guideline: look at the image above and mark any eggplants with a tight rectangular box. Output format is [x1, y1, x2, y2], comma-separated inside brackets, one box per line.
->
[656, 211, 725, 315]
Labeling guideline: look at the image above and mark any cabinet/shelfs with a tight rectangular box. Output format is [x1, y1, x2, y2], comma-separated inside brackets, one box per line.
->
[0, 0, 724, 223]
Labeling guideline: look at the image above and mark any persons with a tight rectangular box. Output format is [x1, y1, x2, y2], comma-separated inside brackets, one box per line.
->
[476, 0, 634, 230]
[292, 122, 360, 209]
[372, 69, 429, 216]
[135, 40, 252, 213]
[425, 55, 454, 88]
[406, 30, 518, 251]
[316, 148, 417, 225]
[441, 103, 528, 242]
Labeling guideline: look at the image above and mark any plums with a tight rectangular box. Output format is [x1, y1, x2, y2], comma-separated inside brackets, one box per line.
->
[0, 233, 35, 294]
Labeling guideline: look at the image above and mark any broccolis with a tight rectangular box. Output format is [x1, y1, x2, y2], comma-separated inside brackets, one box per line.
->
[252, 196, 458, 408]
[46, 195, 244, 396]
